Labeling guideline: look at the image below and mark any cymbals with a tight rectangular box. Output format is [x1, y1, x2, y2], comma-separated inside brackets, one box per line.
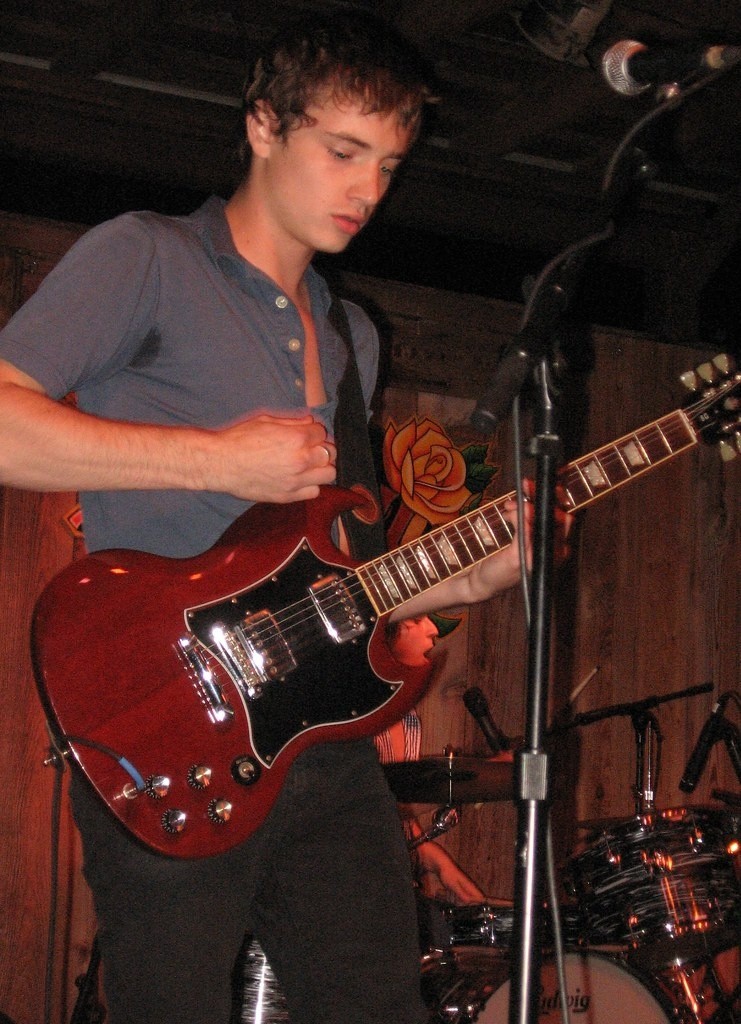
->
[379, 756, 531, 806]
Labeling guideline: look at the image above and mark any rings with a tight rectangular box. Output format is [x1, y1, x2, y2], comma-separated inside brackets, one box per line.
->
[316, 444, 331, 469]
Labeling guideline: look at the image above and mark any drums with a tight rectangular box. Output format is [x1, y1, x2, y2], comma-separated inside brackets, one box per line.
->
[565, 799, 740, 965]
[420, 942, 679, 1023]
[416, 894, 522, 1024]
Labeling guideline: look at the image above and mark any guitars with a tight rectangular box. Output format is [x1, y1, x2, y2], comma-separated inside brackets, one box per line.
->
[25, 353, 740, 858]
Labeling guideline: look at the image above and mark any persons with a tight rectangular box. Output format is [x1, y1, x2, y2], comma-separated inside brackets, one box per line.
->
[0, 19, 579, 1024]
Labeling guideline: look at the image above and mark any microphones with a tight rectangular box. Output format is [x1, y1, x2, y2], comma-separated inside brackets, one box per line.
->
[601, 40, 741, 95]
[679, 694, 729, 794]
[463, 687, 510, 753]
